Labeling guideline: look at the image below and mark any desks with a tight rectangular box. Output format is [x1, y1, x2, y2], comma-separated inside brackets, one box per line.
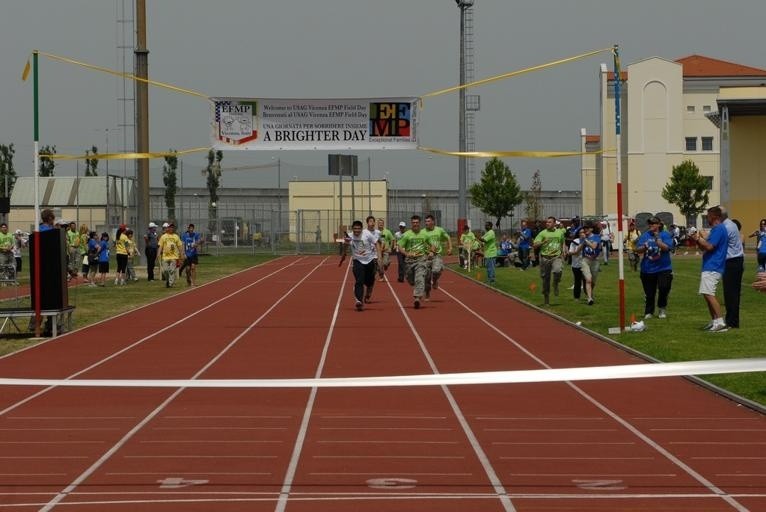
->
[0, 307, 76, 337]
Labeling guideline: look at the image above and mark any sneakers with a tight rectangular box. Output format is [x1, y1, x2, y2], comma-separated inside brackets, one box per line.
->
[709, 323, 728, 333]
[644, 310, 667, 320]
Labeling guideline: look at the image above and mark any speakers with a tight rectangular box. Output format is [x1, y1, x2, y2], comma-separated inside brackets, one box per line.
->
[29, 227, 69, 311]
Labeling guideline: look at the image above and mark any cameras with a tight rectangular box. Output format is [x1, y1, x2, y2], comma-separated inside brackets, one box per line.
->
[689, 226, 699, 235]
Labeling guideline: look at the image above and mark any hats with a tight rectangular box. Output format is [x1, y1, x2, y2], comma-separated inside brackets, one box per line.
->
[148, 223, 158, 228]
[398, 222, 406, 226]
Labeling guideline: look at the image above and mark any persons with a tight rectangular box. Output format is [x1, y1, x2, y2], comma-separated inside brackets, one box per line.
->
[512, 220, 545, 270]
[39, 208, 53, 230]
[559, 216, 613, 304]
[394, 215, 451, 309]
[624, 222, 642, 272]
[479, 221, 497, 284]
[315, 225, 321, 243]
[532, 216, 565, 307]
[635, 217, 674, 319]
[460, 225, 484, 270]
[253, 230, 262, 246]
[0, 220, 203, 287]
[721, 207, 743, 328]
[500, 233, 512, 256]
[690, 207, 729, 331]
[659, 222, 698, 254]
[749, 218, 766, 291]
[347, 221, 382, 310]
[364, 215, 395, 282]
